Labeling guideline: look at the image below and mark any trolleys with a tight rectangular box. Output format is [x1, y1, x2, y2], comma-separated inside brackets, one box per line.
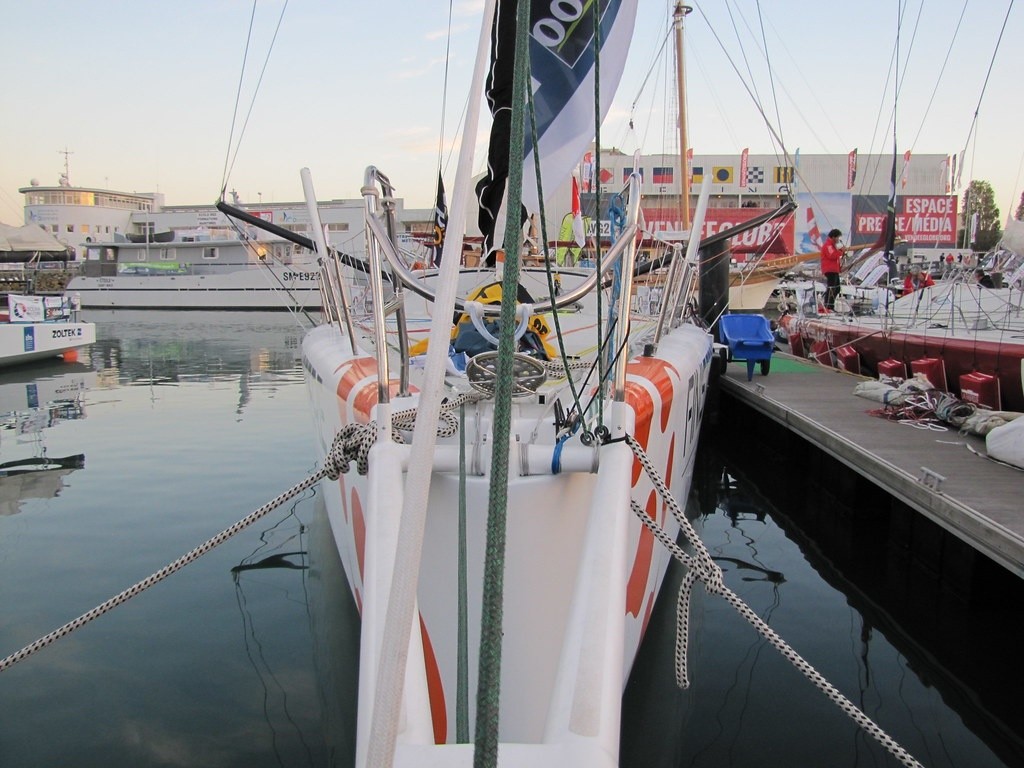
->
[718, 314, 777, 382]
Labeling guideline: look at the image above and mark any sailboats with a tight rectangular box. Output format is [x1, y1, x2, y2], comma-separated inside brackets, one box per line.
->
[217, 2, 715, 768]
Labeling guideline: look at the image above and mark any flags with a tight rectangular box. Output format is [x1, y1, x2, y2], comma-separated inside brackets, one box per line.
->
[572, 178, 586, 249]
[432, 170, 448, 268]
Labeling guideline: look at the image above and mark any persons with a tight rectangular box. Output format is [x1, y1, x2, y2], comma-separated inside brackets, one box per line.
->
[742, 200, 757, 207]
[896, 257, 900, 271]
[822, 229, 847, 313]
[975, 269, 994, 289]
[940, 253, 945, 272]
[901, 265, 935, 297]
[957, 252, 970, 264]
[907, 258, 911, 264]
[946, 253, 954, 265]
[922, 256, 928, 268]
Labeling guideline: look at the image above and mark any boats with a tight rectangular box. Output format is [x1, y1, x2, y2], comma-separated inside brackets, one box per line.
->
[778, 206, 1024, 413]
[0, 223, 97, 368]
[63, 187, 354, 309]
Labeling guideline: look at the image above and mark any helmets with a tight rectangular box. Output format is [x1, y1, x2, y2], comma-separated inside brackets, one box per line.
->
[910, 265, 922, 275]
